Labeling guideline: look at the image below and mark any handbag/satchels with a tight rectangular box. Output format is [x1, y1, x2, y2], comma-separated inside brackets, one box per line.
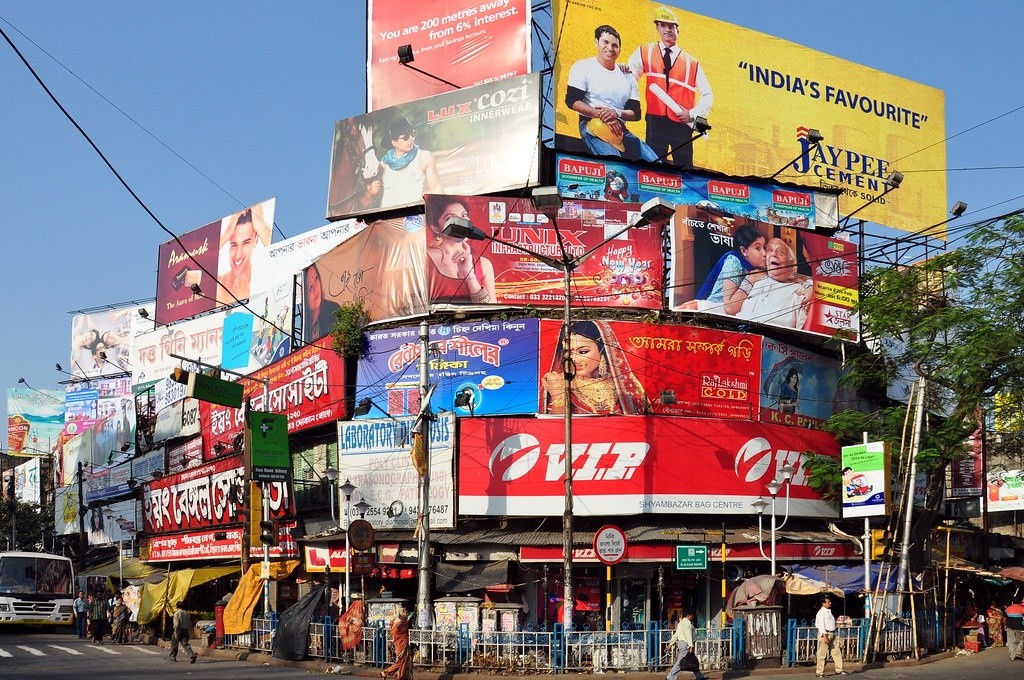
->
[679, 651, 699, 672]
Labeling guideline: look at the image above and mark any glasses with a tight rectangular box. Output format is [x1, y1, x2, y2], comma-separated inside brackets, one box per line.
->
[396, 130, 416, 141]
[106, 331, 110, 344]
[658, 23, 677, 29]
[96, 345, 105, 352]
[97, 594, 102, 596]
[90, 332, 94, 343]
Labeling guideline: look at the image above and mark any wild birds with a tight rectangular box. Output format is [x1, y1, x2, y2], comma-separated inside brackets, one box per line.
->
[330, 665, 344, 674]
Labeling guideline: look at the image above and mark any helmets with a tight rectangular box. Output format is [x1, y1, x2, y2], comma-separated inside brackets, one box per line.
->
[652, 7, 680, 24]
[589, 117, 626, 152]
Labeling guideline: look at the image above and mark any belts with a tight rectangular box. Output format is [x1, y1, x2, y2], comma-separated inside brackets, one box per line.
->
[826, 630, 836, 633]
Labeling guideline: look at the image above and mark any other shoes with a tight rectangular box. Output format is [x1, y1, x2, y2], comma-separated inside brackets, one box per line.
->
[92, 638, 95, 643]
[97, 641, 103, 645]
[380, 670, 387, 680]
[166, 657, 177, 662]
[1009, 657, 1015, 661]
[816, 674, 829, 679]
[190, 654, 197, 664]
[835, 671, 847, 676]
[1016, 654, 1022, 658]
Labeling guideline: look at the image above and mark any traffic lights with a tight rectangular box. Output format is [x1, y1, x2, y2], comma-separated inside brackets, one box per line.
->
[172, 369, 191, 382]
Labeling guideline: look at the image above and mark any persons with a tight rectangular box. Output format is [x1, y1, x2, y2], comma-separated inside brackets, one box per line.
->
[814, 596, 848, 678]
[675, 224, 814, 328]
[565, 25, 662, 163]
[379, 608, 414, 680]
[222, 588, 234, 602]
[71, 309, 132, 377]
[166, 601, 197, 664]
[73, 591, 132, 645]
[617, 8, 714, 165]
[216, 206, 288, 363]
[541, 320, 654, 415]
[602, 177, 629, 203]
[956, 595, 1024, 661]
[88, 507, 108, 545]
[425, 196, 497, 305]
[301, 263, 344, 346]
[665, 610, 709, 680]
[359, 116, 446, 211]
[116, 405, 131, 456]
[0, 569, 19, 586]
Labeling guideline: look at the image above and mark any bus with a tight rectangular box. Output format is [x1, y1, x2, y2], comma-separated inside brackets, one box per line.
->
[0, 551, 75, 626]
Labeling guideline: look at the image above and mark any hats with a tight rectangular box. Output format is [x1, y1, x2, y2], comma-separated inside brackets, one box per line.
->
[381, 117, 427, 149]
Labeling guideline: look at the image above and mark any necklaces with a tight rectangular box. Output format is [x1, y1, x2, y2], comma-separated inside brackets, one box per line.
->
[572, 376, 616, 414]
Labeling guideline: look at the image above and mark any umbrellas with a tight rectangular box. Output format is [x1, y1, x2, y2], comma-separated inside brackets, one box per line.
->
[994, 565, 1024, 596]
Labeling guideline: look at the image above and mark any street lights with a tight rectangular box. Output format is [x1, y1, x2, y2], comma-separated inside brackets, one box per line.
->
[440, 183, 678, 643]
[354, 495, 370, 520]
[338, 476, 357, 611]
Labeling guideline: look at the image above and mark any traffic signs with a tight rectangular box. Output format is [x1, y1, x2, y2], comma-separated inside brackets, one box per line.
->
[675, 545, 708, 570]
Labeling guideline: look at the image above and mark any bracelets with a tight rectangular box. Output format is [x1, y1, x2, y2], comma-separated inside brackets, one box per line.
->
[469, 286, 490, 304]
[615, 108, 622, 117]
